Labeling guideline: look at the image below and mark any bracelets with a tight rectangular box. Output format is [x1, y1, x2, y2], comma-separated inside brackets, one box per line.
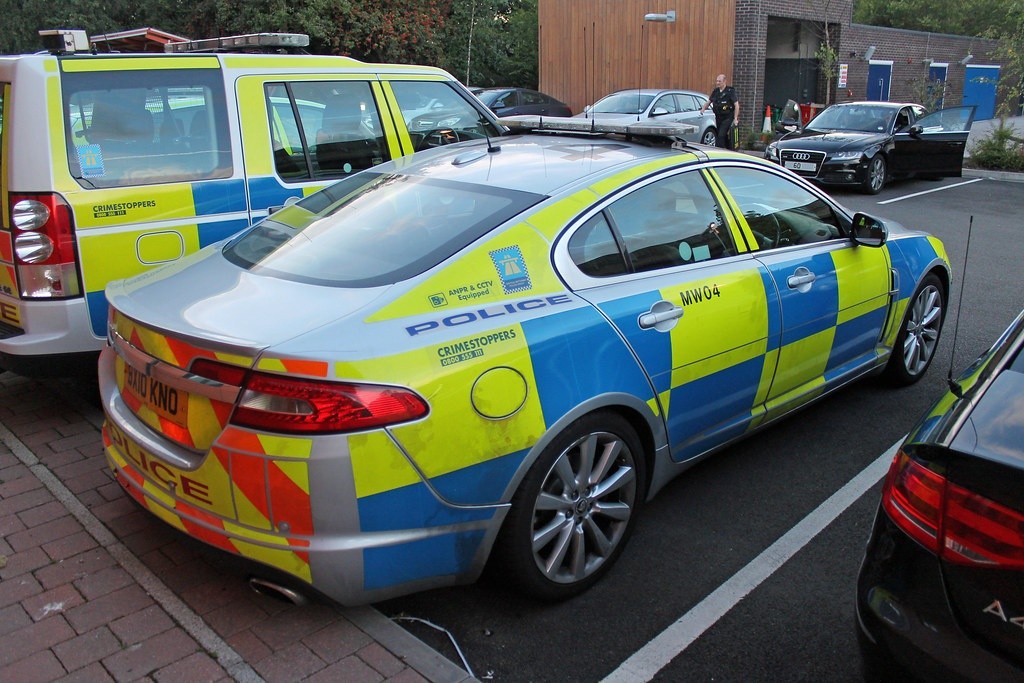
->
[733, 118, 738, 120]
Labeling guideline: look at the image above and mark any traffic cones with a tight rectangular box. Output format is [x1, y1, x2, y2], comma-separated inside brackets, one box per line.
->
[760, 105, 771, 141]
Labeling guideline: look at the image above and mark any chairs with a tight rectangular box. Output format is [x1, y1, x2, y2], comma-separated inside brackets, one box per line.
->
[90, 89, 159, 167]
[641, 190, 723, 257]
[316, 94, 379, 170]
[181, 110, 213, 160]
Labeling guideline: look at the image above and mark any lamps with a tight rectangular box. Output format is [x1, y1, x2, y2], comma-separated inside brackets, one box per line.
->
[957, 54, 973, 65]
[850, 46, 876, 61]
[645, 10, 676, 23]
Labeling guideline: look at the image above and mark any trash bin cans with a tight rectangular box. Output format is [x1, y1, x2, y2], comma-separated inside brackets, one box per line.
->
[800, 103, 816, 125]
[763, 109, 779, 122]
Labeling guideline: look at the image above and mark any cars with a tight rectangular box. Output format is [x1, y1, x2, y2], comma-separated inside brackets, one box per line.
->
[853, 304, 1024, 683]
[91, 130, 958, 610]
[569, 87, 718, 147]
[762, 99, 980, 195]
[401, 85, 574, 139]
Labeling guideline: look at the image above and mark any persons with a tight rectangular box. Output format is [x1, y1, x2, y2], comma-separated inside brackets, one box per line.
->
[700, 74, 740, 150]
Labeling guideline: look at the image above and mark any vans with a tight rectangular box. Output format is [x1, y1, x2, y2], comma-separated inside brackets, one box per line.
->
[0, 30, 515, 381]
